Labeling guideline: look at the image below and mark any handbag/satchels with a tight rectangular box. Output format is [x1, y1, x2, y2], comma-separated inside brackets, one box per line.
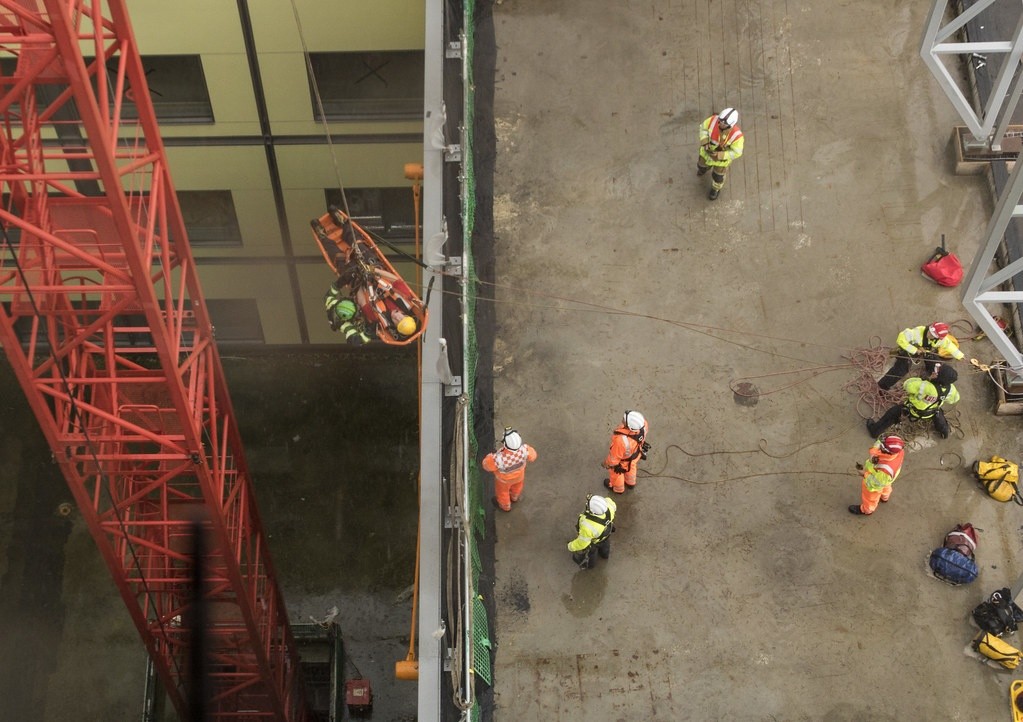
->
[972, 455, 1023, 507]
[943, 523, 984, 557]
[972, 587, 1023, 638]
[929, 547, 978, 586]
[972, 631, 1023, 671]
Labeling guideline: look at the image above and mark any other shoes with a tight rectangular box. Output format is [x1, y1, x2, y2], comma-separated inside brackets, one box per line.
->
[709, 189, 719, 200]
[939, 426, 948, 439]
[697, 170, 706, 176]
[625, 481, 635, 489]
[598, 549, 609, 559]
[880, 498, 888, 503]
[867, 418, 879, 438]
[604, 479, 624, 495]
[573, 552, 594, 569]
[848, 505, 872, 515]
[491, 496, 517, 513]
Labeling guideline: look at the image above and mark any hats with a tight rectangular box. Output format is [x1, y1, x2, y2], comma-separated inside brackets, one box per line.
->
[937, 364, 958, 386]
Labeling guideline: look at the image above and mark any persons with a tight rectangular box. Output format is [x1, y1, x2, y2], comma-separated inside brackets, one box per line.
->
[309, 206, 417, 336]
[600, 409, 648, 495]
[482, 428, 537, 511]
[695, 107, 745, 201]
[847, 431, 905, 515]
[866, 362, 961, 440]
[877, 321, 966, 402]
[567, 494, 616, 569]
[323, 266, 383, 348]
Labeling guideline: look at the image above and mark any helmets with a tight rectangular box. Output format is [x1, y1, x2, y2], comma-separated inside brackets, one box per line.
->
[623, 411, 645, 431]
[501, 429, 522, 452]
[883, 435, 905, 455]
[928, 321, 949, 340]
[588, 495, 608, 515]
[397, 316, 417, 336]
[717, 107, 738, 129]
[335, 299, 356, 321]
